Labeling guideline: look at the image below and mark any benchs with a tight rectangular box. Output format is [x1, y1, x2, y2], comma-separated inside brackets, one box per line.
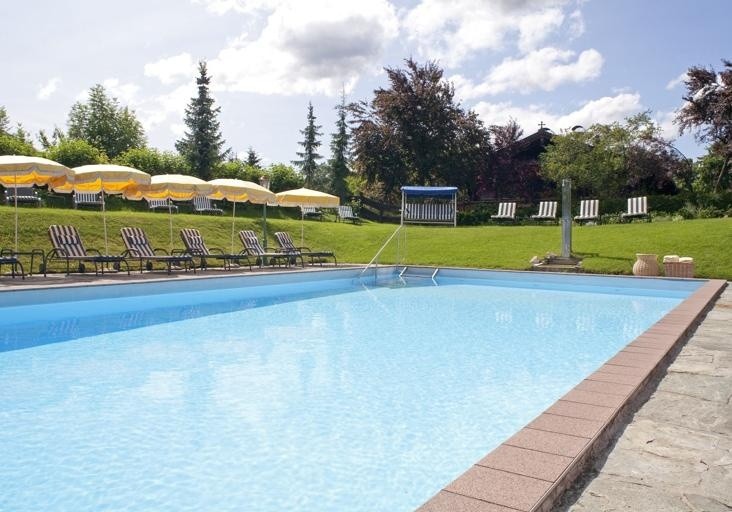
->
[72, 191, 107, 211]
[192, 194, 224, 215]
[299, 206, 324, 221]
[4, 185, 42, 208]
[335, 205, 359, 224]
[148, 197, 178, 214]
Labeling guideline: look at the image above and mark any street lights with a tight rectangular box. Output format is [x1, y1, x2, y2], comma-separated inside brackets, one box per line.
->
[257, 173, 271, 266]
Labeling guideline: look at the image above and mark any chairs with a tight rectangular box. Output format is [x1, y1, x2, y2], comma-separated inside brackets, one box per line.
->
[1, 247, 49, 279]
[272, 231, 339, 269]
[489, 195, 653, 223]
[236, 229, 305, 270]
[176, 226, 248, 272]
[42, 222, 131, 276]
[113, 224, 197, 275]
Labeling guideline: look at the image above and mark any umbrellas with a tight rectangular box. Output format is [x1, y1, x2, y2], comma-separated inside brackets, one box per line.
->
[124, 172, 212, 266]
[268, 188, 339, 259]
[206, 178, 277, 262]
[52, 164, 152, 268]
[0, 153, 77, 270]
